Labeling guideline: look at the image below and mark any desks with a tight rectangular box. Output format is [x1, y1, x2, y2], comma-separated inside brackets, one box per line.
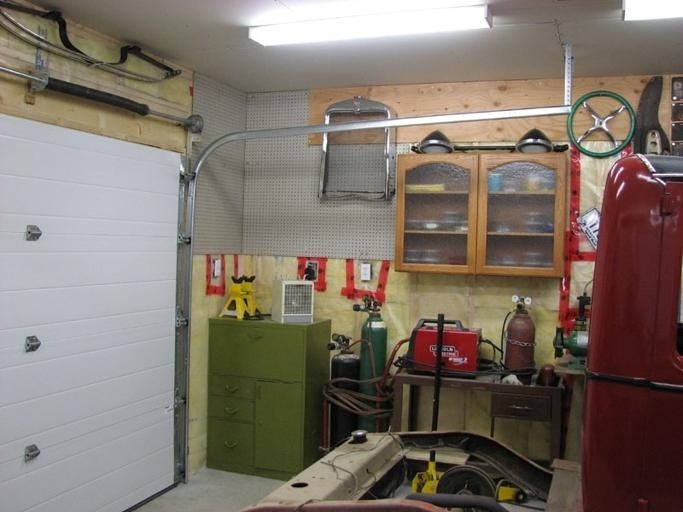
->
[388, 373, 565, 463]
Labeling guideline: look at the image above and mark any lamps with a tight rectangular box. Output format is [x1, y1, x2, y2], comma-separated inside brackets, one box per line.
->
[620, 1, 682, 23]
[413, 129, 569, 154]
[246, 5, 496, 48]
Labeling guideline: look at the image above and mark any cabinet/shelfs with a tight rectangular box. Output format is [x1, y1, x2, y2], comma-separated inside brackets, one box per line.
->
[392, 151, 571, 278]
[205, 314, 331, 484]
